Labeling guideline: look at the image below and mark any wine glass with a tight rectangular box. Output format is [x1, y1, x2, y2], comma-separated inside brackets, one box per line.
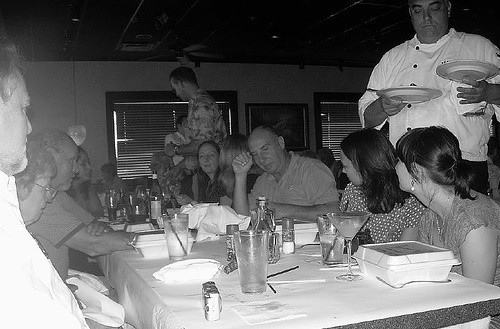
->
[326, 211, 372, 280]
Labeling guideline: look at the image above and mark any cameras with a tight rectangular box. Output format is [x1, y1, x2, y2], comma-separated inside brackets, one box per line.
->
[201, 281, 223, 321]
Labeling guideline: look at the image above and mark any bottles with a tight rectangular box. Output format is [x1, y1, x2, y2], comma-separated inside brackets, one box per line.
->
[249, 197, 273, 235]
[251, 197, 276, 231]
[226, 225, 239, 261]
[202, 281, 221, 322]
[148, 170, 162, 224]
[281, 216, 295, 254]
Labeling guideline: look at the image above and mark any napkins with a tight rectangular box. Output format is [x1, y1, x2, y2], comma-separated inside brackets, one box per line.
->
[151, 258, 222, 283]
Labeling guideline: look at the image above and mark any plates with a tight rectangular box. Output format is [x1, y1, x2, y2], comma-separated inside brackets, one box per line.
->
[376, 86, 443, 103]
[435, 59, 500, 82]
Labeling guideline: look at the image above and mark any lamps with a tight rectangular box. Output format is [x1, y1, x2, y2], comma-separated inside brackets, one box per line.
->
[338, 63, 345, 73]
[194, 57, 201, 69]
[71, 6, 81, 22]
[65, 49, 87, 147]
[298, 63, 305, 71]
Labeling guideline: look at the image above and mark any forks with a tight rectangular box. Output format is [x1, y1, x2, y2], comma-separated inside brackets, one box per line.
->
[377, 276, 452, 289]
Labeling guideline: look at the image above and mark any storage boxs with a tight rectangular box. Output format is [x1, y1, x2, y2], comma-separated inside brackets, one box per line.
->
[126, 229, 197, 258]
[351, 239, 462, 289]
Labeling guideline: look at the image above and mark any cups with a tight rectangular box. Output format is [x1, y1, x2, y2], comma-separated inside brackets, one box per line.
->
[268, 233, 281, 264]
[234, 230, 268, 295]
[163, 214, 189, 260]
[318, 215, 337, 263]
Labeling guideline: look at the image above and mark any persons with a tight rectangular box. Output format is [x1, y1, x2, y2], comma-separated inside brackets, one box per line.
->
[98, 163, 121, 193]
[358, 0, 500, 197]
[13, 144, 58, 227]
[487, 136, 500, 197]
[175, 141, 221, 208]
[339, 128, 426, 244]
[26, 127, 135, 309]
[395, 125, 500, 286]
[165, 66, 227, 203]
[205, 133, 260, 206]
[65, 147, 102, 212]
[0, 42, 90, 329]
[154, 114, 194, 199]
[301, 147, 351, 189]
[232, 124, 339, 221]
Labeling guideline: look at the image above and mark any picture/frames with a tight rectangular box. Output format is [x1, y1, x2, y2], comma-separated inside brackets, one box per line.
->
[244, 102, 310, 152]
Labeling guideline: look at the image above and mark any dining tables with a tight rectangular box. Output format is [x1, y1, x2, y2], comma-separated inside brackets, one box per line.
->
[85, 213, 500, 329]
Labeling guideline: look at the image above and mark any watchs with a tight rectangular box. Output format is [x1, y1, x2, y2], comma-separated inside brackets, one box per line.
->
[174, 146, 179, 155]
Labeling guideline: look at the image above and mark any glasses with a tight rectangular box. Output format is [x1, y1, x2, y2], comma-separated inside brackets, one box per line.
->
[34, 182, 57, 202]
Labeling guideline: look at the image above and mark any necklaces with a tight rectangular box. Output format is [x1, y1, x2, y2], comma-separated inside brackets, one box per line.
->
[436, 196, 450, 235]
